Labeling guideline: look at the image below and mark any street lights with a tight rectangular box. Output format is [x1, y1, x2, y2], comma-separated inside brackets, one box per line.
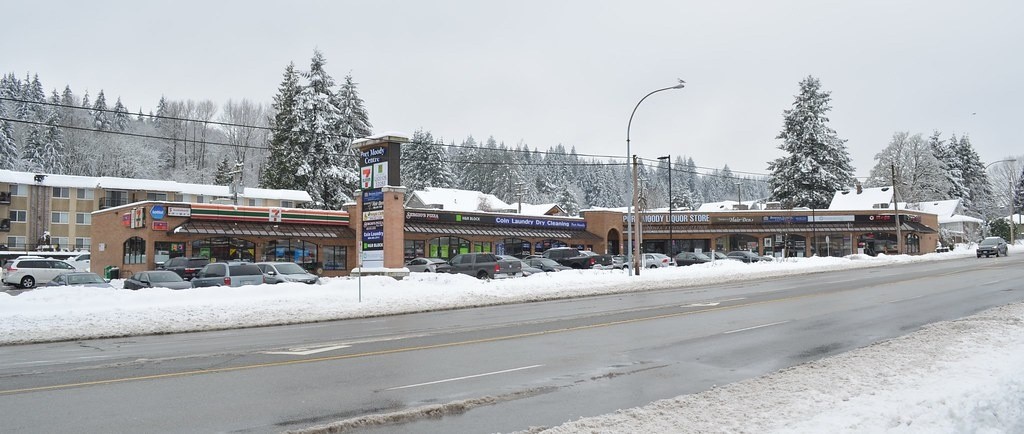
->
[803, 181, 819, 256]
[657, 154, 672, 262]
[982, 159, 1016, 239]
[627, 84, 685, 276]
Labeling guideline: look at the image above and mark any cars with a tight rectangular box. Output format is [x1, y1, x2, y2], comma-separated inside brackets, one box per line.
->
[977, 236, 1008, 258]
[46, 272, 116, 291]
[405, 256, 451, 274]
[773, 240, 841, 257]
[632, 250, 773, 269]
[189, 261, 264, 288]
[1, 251, 90, 290]
[447, 247, 628, 280]
[255, 261, 319, 285]
[123, 270, 192, 291]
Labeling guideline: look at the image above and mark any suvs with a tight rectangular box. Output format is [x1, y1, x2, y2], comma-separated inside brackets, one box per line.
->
[161, 256, 209, 281]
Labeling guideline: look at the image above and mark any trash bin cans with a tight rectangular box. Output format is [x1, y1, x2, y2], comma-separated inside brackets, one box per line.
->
[103, 265, 119, 281]
